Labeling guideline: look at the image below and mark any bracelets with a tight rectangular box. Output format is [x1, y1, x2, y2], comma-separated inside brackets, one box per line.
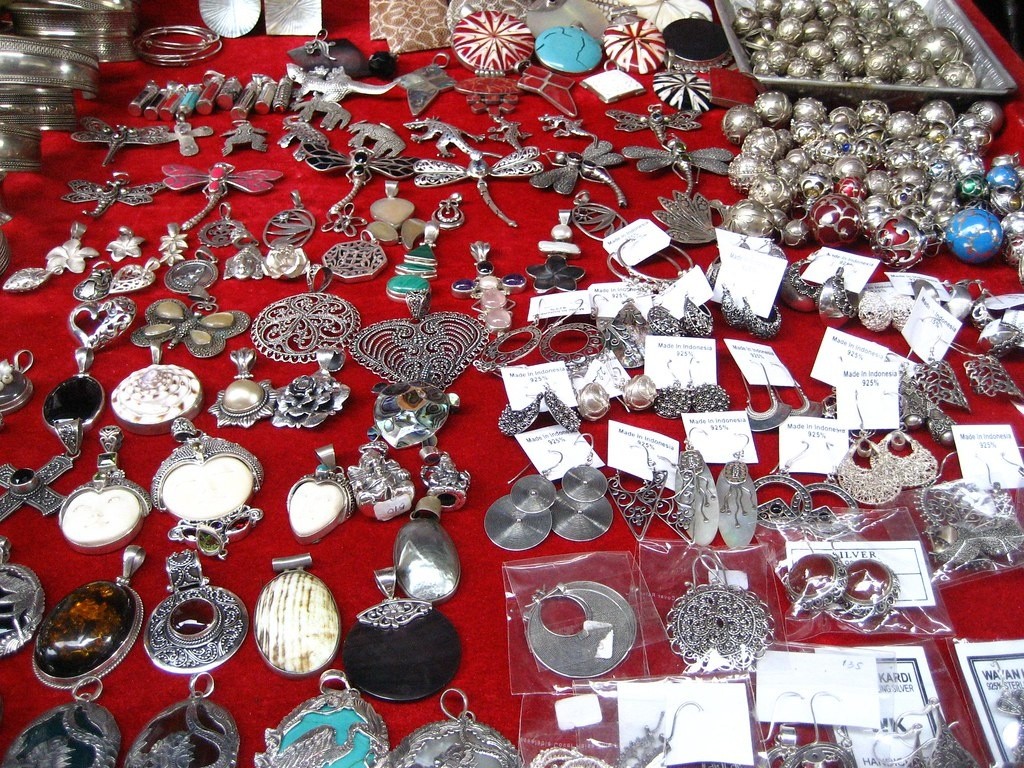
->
[0, 0, 223, 273]
